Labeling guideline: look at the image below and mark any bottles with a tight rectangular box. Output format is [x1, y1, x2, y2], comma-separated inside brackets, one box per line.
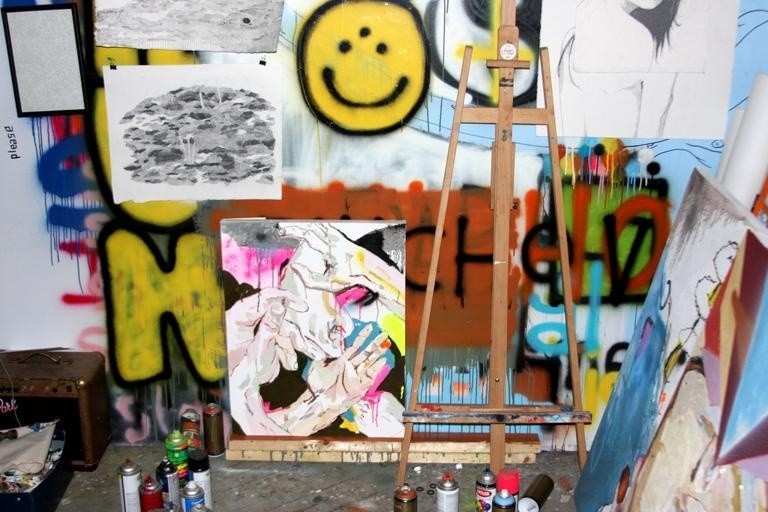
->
[389, 466, 555, 512]
[118, 400, 223, 512]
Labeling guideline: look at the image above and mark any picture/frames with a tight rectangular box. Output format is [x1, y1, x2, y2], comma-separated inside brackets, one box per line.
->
[1, 3, 88, 117]
